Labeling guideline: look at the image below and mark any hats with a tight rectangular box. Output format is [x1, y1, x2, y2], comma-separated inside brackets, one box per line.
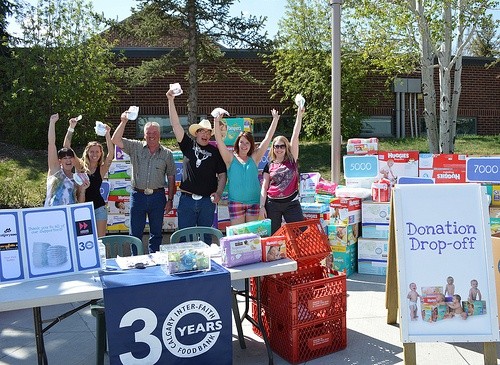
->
[189, 119, 215, 136]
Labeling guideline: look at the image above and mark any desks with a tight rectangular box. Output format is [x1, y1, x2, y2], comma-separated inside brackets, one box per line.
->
[0, 245, 297, 365]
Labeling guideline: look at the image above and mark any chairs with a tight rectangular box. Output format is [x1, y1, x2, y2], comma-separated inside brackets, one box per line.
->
[90, 235, 144, 365]
[169, 226, 247, 350]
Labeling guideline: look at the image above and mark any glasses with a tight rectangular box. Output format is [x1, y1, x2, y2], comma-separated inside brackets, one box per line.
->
[199, 129, 210, 134]
[274, 144, 285, 149]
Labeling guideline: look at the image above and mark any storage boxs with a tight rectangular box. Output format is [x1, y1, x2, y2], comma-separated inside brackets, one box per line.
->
[99, 118, 500, 365]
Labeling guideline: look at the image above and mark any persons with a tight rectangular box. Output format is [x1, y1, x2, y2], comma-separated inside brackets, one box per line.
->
[267, 246, 285, 262]
[44, 112, 91, 206]
[443, 276, 455, 296]
[115, 200, 130, 215]
[379, 159, 397, 183]
[110, 104, 177, 257]
[444, 294, 468, 319]
[468, 279, 482, 305]
[330, 207, 340, 224]
[62, 115, 114, 238]
[210, 107, 281, 225]
[406, 283, 424, 322]
[258, 93, 306, 236]
[164, 81, 228, 246]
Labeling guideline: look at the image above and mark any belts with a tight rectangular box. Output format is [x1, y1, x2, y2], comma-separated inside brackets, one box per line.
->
[133, 187, 164, 195]
[181, 192, 209, 200]
